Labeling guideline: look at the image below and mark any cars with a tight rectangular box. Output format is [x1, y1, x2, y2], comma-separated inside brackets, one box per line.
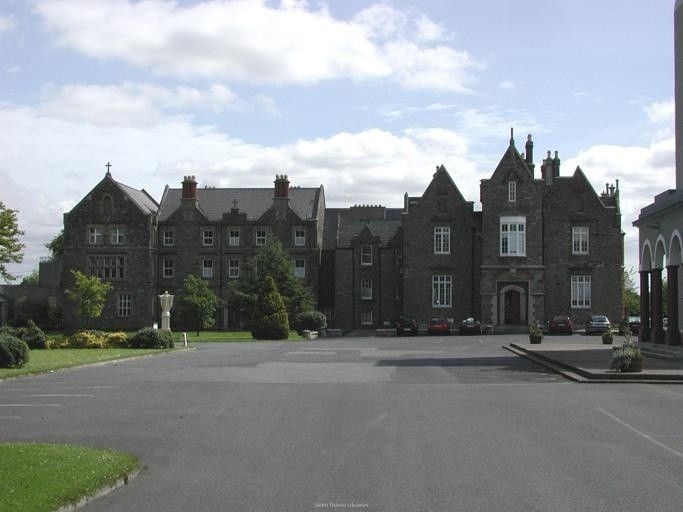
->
[618, 316, 668, 336]
[584, 315, 612, 336]
[546, 315, 573, 335]
[459, 314, 480, 334]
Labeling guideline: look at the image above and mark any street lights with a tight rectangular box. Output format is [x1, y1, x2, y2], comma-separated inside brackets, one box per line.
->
[157, 291, 174, 332]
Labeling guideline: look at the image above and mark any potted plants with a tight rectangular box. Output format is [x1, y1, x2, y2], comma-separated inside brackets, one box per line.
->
[602, 328, 643, 371]
[528, 323, 542, 344]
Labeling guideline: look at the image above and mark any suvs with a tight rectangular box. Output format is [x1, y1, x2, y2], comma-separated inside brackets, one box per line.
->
[426, 314, 449, 336]
[396, 313, 418, 336]
